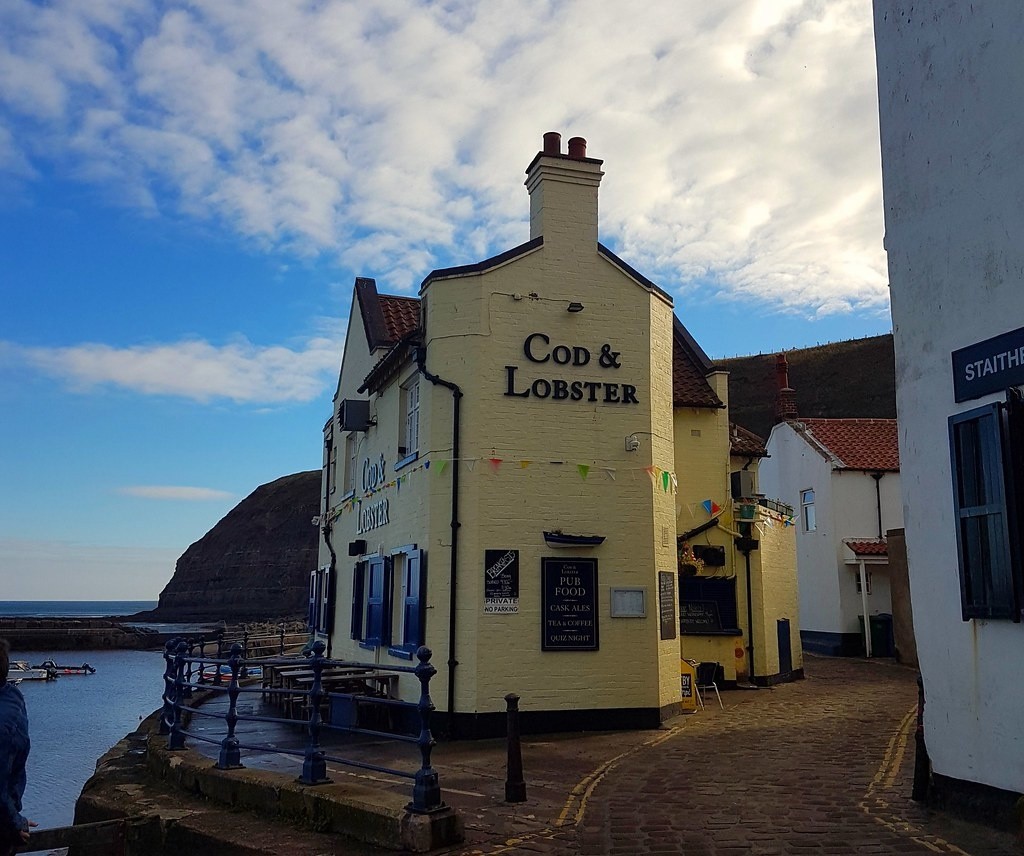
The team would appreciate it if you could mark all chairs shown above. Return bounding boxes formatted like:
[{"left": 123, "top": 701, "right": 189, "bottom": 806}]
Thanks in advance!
[{"left": 693, "top": 661, "right": 725, "bottom": 711}]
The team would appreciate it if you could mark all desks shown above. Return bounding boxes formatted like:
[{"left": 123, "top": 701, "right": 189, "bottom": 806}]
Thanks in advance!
[
  {"left": 295, "top": 670, "right": 400, "bottom": 737},
  {"left": 262, "top": 663, "right": 280, "bottom": 700},
  {"left": 273, "top": 664, "right": 311, "bottom": 705},
  {"left": 279, "top": 667, "right": 372, "bottom": 719}
]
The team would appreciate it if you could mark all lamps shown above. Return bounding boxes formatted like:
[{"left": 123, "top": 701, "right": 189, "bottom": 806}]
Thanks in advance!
[{"left": 567, "top": 301, "right": 584, "bottom": 313}]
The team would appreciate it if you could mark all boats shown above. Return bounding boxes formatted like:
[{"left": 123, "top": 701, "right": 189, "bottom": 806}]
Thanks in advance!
[
  {"left": 199, "top": 666, "right": 263, "bottom": 682},
  {"left": 7, "top": 663, "right": 59, "bottom": 685},
  {"left": 7, "top": 658, "right": 96, "bottom": 676}
]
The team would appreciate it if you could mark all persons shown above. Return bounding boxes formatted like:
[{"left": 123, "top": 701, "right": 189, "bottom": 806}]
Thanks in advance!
[{"left": 0, "top": 640, "right": 38, "bottom": 856}]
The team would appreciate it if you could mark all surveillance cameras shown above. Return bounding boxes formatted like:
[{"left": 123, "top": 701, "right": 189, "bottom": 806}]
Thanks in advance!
[{"left": 631, "top": 441, "right": 640, "bottom": 449}]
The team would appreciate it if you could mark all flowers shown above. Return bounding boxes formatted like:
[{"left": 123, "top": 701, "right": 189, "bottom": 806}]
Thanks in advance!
[{"left": 681, "top": 551, "right": 705, "bottom": 574}]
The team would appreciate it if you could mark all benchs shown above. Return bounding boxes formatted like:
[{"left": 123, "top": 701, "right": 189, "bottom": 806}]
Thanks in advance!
[{"left": 258, "top": 674, "right": 403, "bottom": 710}]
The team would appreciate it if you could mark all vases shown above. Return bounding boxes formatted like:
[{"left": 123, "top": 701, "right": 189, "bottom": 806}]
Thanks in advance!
[{"left": 684, "top": 564, "right": 698, "bottom": 576}]
